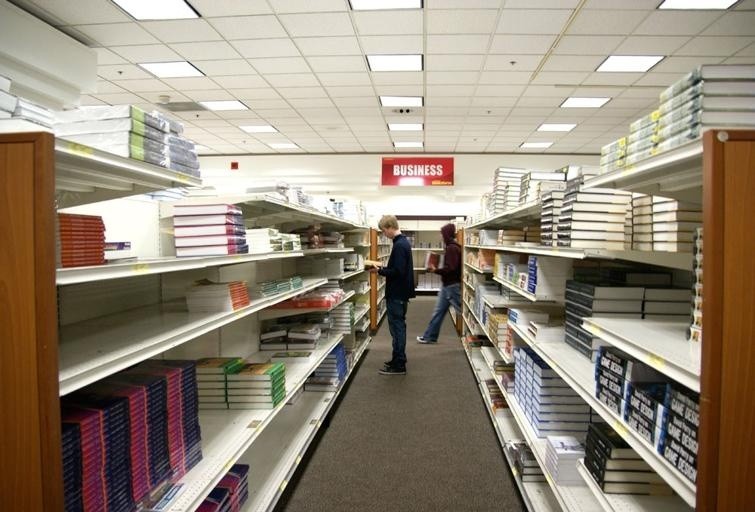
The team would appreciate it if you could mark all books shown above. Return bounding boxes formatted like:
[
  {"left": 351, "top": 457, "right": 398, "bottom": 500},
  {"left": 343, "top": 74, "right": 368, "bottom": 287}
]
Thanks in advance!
[
  {"left": 632, "top": 194, "right": 652, "bottom": 250},
  {"left": 564, "top": 260, "right": 690, "bottom": 364},
  {"left": 244, "top": 227, "right": 302, "bottom": 256},
  {"left": 557, "top": 188, "right": 634, "bottom": 250},
  {"left": 685, "top": 225, "right": 703, "bottom": 345},
  {"left": 546, "top": 435, "right": 585, "bottom": 488},
  {"left": 491, "top": 252, "right": 572, "bottom": 298},
  {"left": 59, "top": 358, "right": 204, "bottom": 511},
  {"left": 56, "top": 213, "right": 139, "bottom": 269},
  {"left": 581, "top": 424, "right": 673, "bottom": 495},
  {"left": 462, "top": 287, "right": 563, "bottom": 346},
  {"left": 488, "top": 166, "right": 565, "bottom": 249},
  {"left": 364, "top": 260, "right": 383, "bottom": 272},
  {"left": 173, "top": 204, "right": 249, "bottom": 259},
  {"left": 506, "top": 439, "right": 547, "bottom": 482},
  {"left": 554, "top": 167, "right": 598, "bottom": 189},
  {"left": 654, "top": 65, "right": 754, "bottom": 153},
  {"left": 295, "top": 218, "right": 367, "bottom": 300},
  {"left": 194, "top": 464, "right": 249, "bottom": 512},
  {"left": 425, "top": 250, "right": 444, "bottom": 273},
  {"left": 468, "top": 348, "right": 516, "bottom": 412},
  {"left": 465, "top": 214, "right": 492, "bottom": 287},
  {"left": 512, "top": 346, "right": 606, "bottom": 438},
  {"left": 594, "top": 345, "right": 632, "bottom": 420},
  {"left": 624, "top": 379, "right": 699, "bottom": 487},
  {"left": 596, "top": 137, "right": 623, "bottom": 174},
  {"left": 260, "top": 301, "right": 356, "bottom": 392},
  {"left": 197, "top": 355, "right": 286, "bottom": 410},
  {"left": 625, "top": 109, "right": 659, "bottom": 165},
  {"left": 650, "top": 193, "right": 702, "bottom": 251},
  {"left": 186, "top": 263, "right": 301, "bottom": 313},
  {"left": 1, "top": 73, "right": 200, "bottom": 182}
]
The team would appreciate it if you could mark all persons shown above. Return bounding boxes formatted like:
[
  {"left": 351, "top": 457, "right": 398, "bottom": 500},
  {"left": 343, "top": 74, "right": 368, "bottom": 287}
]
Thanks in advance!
[
  {"left": 368, "top": 214, "right": 418, "bottom": 374},
  {"left": 417, "top": 224, "right": 461, "bottom": 344}
]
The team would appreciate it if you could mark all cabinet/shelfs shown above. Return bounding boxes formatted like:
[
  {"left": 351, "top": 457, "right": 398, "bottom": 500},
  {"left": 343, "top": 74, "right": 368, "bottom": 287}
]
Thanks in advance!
[
  {"left": 0, "top": 132, "right": 373, "bottom": 512},
  {"left": 372, "top": 229, "right": 393, "bottom": 333},
  {"left": 448, "top": 227, "right": 461, "bottom": 336},
  {"left": 400, "top": 229, "right": 446, "bottom": 296},
  {"left": 462, "top": 129, "right": 755, "bottom": 512}
]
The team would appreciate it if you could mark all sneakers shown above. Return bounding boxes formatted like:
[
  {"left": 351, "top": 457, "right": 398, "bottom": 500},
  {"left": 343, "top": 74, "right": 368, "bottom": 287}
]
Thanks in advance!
[
  {"left": 417, "top": 335, "right": 438, "bottom": 344},
  {"left": 384, "top": 358, "right": 408, "bottom": 365},
  {"left": 378, "top": 367, "right": 407, "bottom": 374}
]
